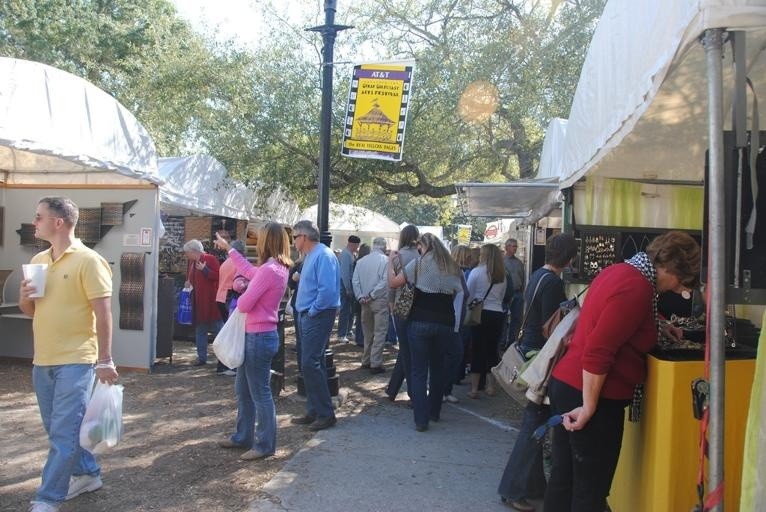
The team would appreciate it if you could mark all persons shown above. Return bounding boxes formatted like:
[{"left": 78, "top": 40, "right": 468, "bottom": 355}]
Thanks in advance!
[
  {"left": 544, "top": 230, "right": 701, "bottom": 512},
  {"left": 497, "top": 233, "right": 579, "bottom": 511},
  {"left": 213, "top": 221, "right": 294, "bottom": 461},
  {"left": 388, "top": 233, "right": 462, "bottom": 430},
  {"left": 657, "top": 269, "right": 707, "bottom": 328},
  {"left": 18, "top": 194, "right": 119, "bottom": 512},
  {"left": 183, "top": 238, "right": 225, "bottom": 367},
  {"left": 290, "top": 220, "right": 340, "bottom": 431},
  {"left": 288, "top": 219, "right": 525, "bottom": 410},
  {"left": 215, "top": 240, "right": 247, "bottom": 377}
]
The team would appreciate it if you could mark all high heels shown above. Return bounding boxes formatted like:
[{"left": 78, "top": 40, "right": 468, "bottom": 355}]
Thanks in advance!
[{"left": 501, "top": 496, "right": 536, "bottom": 511}]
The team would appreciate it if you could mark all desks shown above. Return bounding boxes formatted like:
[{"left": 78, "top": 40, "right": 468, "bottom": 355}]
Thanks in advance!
[{"left": 603, "top": 338, "right": 756, "bottom": 510}]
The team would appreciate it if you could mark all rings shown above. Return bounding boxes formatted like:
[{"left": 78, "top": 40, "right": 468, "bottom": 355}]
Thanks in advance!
[{"left": 571, "top": 428, "right": 573, "bottom": 433}]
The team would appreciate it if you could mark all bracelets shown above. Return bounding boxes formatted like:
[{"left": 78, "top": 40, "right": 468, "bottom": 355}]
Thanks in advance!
[{"left": 97, "top": 358, "right": 113, "bottom": 363}]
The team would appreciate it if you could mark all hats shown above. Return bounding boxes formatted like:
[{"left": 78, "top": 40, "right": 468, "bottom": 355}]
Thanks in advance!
[{"left": 348, "top": 236, "right": 361, "bottom": 244}]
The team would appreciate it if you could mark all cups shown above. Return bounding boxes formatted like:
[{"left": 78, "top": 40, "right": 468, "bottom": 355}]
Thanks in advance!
[{"left": 22, "top": 263, "right": 48, "bottom": 298}]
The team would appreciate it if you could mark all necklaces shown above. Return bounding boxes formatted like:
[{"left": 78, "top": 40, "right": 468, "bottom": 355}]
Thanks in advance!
[{"left": 678, "top": 279, "right": 695, "bottom": 301}]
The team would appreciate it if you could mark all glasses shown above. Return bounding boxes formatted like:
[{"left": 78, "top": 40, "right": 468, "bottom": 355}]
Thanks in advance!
[
  {"left": 37, "top": 214, "right": 59, "bottom": 221},
  {"left": 534, "top": 415, "right": 562, "bottom": 440},
  {"left": 292, "top": 234, "right": 302, "bottom": 240}
]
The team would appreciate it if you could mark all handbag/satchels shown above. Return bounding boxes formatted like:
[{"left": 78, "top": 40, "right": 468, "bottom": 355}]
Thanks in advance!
[
  {"left": 491, "top": 341, "right": 529, "bottom": 408},
  {"left": 393, "top": 282, "right": 415, "bottom": 320},
  {"left": 178, "top": 288, "right": 194, "bottom": 325},
  {"left": 462, "top": 298, "right": 483, "bottom": 326},
  {"left": 542, "top": 299, "right": 576, "bottom": 339},
  {"left": 224, "top": 289, "right": 239, "bottom": 318}
]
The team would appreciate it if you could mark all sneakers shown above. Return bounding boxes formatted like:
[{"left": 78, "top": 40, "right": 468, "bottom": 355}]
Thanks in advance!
[
  {"left": 217, "top": 440, "right": 261, "bottom": 460},
  {"left": 293, "top": 416, "right": 336, "bottom": 430},
  {"left": 217, "top": 370, "right": 236, "bottom": 376},
  {"left": 65, "top": 476, "right": 103, "bottom": 500},
  {"left": 30, "top": 501, "right": 62, "bottom": 512},
  {"left": 338, "top": 337, "right": 479, "bottom": 432}
]
